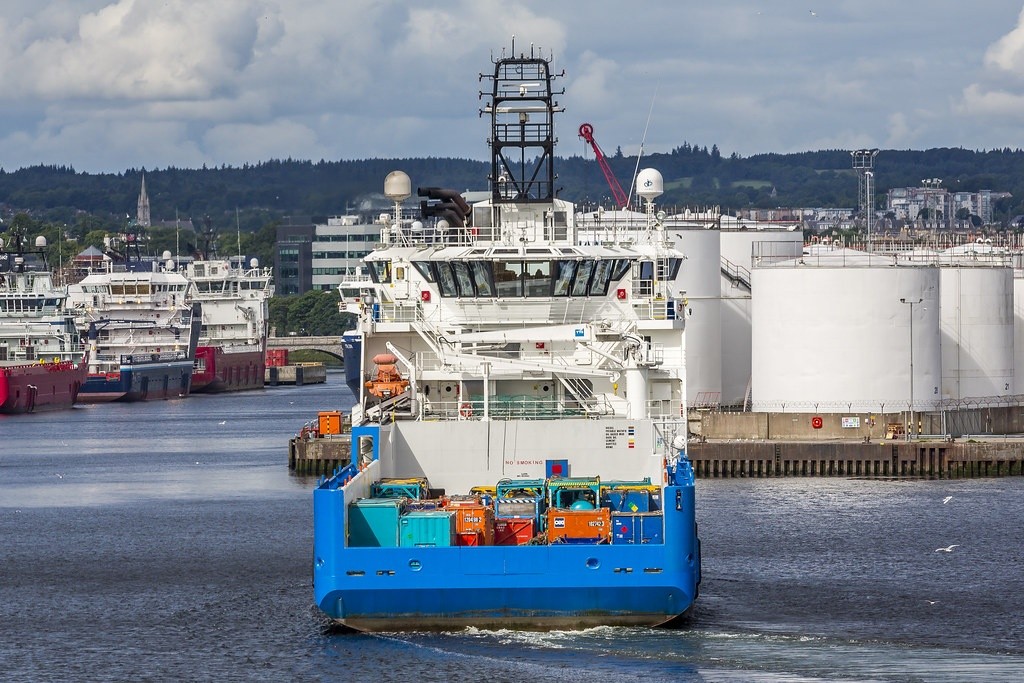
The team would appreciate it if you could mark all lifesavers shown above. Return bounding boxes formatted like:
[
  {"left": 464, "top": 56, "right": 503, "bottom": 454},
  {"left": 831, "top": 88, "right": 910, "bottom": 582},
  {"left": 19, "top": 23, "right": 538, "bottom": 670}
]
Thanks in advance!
[
  {"left": 459, "top": 403, "right": 474, "bottom": 418},
  {"left": 812, "top": 418, "right": 822, "bottom": 430}
]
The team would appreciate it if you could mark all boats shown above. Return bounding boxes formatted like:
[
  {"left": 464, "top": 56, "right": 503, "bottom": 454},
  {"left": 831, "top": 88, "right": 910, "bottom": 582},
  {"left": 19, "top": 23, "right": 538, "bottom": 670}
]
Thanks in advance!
[
  {"left": 312, "top": 169, "right": 700, "bottom": 630},
  {"left": 0, "top": 222, "right": 275, "bottom": 416}
]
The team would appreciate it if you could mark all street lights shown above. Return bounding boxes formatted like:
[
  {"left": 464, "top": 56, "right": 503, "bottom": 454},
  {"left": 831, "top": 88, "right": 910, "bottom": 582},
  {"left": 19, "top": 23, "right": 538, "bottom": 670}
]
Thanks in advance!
[
  {"left": 865, "top": 171, "right": 875, "bottom": 267},
  {"left": 899, "top": 298, "right": 924, "bottom": 437}
]
